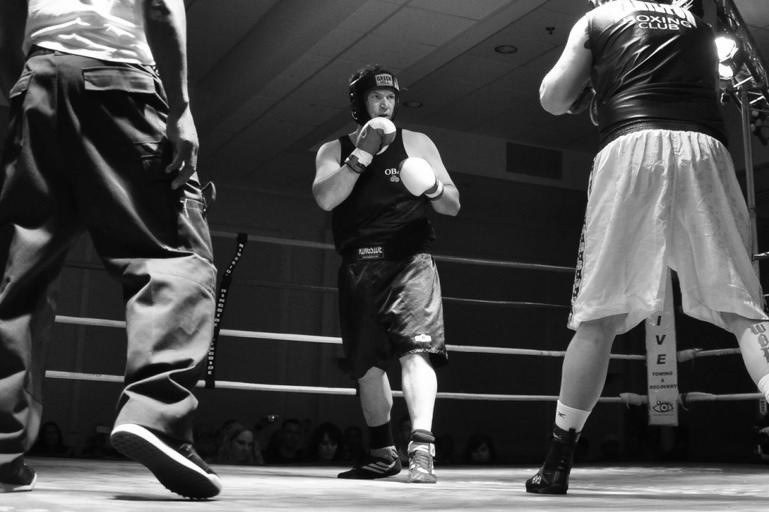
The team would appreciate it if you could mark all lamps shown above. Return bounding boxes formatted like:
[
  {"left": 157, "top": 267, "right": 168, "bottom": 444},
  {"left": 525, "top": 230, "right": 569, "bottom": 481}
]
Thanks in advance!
[{"left": 713, "top": 20, "right": 743, "bottom": 82}]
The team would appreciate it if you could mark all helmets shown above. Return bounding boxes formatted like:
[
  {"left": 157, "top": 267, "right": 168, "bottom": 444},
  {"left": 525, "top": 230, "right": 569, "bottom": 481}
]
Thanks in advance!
[{"left": 348, "top": 69, "right": 403, "bottom": 126}]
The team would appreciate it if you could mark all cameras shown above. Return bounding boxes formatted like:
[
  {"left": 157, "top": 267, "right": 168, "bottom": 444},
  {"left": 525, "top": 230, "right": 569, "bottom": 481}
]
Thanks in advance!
[{"left": 265, "top": 412, "right": 280, "bottom": 424}]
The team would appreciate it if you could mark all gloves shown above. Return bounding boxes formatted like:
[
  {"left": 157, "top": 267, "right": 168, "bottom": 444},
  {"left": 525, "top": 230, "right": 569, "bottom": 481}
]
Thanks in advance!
[
  {"left": 342, "top": 117, "right": 397, "bottom": 175},
  {"left": 397, "top": 157, "right": 444, "bottom": 202}
]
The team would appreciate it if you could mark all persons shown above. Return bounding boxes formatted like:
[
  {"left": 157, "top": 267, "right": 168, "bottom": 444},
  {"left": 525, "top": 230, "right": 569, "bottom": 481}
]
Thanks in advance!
[
  {"left": 524, "top": 2, "right": 769, "bottom": 498},
  {"left": 308, "top": 63, "right": 464, "bottom": 483},
  {"left": 32, "top": 410, "right": 514, "bottom": 466},
  {"left": 1, "top": 0, "right": 224, "bottom": 500}
]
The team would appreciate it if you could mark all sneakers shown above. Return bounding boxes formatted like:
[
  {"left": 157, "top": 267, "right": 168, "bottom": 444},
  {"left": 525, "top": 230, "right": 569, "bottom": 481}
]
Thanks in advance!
[
  {"left": 526, "top": 463, "right": 571, "bottom": 494},
  {"left": 338, "top": 443, "right": 401, "bottom": 479},
  {"left": 109, "top": 422, "right": 223, "bottom": 499},
  {"left": 407, "top": 440, "right": 438, "bottom": 484}
]
